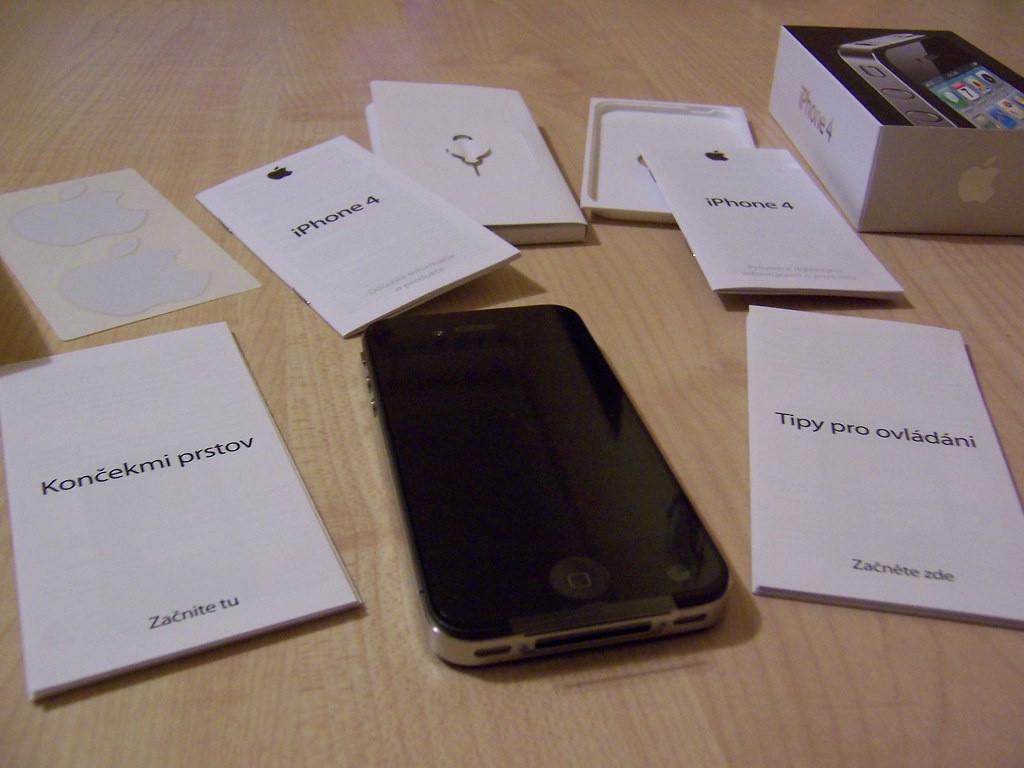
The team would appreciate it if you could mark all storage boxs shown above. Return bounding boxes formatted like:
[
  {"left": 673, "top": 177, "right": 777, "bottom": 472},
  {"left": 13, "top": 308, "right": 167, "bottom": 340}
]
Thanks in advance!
[{"left": 766, "top": 23, "right": 1024, "bottom": 237}]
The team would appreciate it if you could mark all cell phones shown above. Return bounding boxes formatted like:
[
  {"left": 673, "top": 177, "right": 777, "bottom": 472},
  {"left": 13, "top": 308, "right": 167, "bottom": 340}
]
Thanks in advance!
[
  {"left": 360, "top": 306, "right": 729, "bottom": 666},
  {"left": 839, "top": 32, "right": 1024, "bottom": 130}
]
